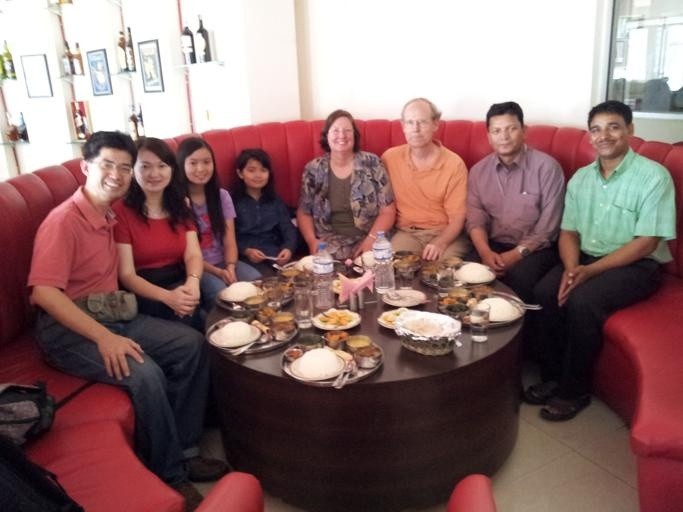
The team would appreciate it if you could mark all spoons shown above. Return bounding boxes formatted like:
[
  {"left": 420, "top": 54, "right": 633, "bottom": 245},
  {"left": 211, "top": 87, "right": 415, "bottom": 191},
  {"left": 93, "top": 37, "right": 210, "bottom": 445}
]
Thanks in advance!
[
  {"left": 230, "top": 333, "right": 272, "bottom": 358},
  {"left": 333, "top": 357, "right": 355, "bottom": 390}
]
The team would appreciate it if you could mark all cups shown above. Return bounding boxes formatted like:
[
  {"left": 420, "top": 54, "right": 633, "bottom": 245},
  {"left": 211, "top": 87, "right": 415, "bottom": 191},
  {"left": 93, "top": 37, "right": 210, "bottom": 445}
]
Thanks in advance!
[
  {"left": 361, "top": 250, "right": 372, "bottom": 273},
  {"left": 290, "top": 280, "right": 315, "bottom": 328},
  {"left": 467, "top": 300, "right": 489, "bottom": 343},
  {"left": 396, "top": 260, "right": 415, "bottom": 290}
]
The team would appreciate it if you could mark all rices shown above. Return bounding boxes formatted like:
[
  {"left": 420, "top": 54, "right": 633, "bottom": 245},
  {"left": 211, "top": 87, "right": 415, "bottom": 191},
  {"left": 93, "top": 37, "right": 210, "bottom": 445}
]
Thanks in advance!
[
  {"left": 216, "top": 322, "right": 252, "bottom": 344},
  {"left": 480, "top": 297, "right": 518, "bottom": 322},
  {"left": 455, "top": 263, "right": 491, "bottom": 283},
  {"left": 297, "top": 255, "right": 315, "bottom": 270},
  {"left": 223, "top": 282, "right": 257, "bottom": 301},
  {"left": 294, "top": 348, "right": 337, "bottom": 379}
]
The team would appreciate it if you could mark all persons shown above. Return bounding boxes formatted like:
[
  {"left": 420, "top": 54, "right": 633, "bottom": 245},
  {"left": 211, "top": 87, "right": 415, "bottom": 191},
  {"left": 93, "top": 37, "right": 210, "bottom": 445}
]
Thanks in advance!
[
  {"left": 108, "top": 136, "right": 205, "bottom": 339},
  {"left": 380, "top": 98, "right": 474, "bottom": 260},
  {"left": 174, "top": 138, "right": 263, "bottom": 311},
  {"left": 26, "top": 131, "right": 229, "bottom": 511},
  {"left": 464, "top": 102, "right": 564, "bottom": 299},
  {"left": 295, "top": 108, "right": 397, "bottom": 261},
  {"left": 522, "top": 100, "right": 680, "bottom": 423},
  {"left": 228, "top": 148, "right": 299, "bottom": 277}
]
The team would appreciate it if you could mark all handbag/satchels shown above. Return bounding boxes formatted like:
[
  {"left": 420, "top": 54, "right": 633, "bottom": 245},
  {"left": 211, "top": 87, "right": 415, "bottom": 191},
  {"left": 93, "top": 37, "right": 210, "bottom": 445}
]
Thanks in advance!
[{"left": 73, "top": 291, "right": 140, "bottom": 322}]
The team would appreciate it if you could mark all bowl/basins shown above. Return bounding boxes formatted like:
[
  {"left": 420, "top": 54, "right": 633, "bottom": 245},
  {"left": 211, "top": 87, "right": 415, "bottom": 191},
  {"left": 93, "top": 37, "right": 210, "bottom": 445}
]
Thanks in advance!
[
  {"left": 422, "top": 255, "right": 458, "bottom": 287},
  {"left": 344, "top": 334, "right": 371, "bottom": 353},
  {"left": 285, "top": 346, "right": 301, "bottom": 363},
  {"left": 390, "top": 249, "right": 419, "bottom": 271},
  {"left": 247, "top": 274, "right": 289, "bottom": 310},
  {"left": 320, "top": 330, "right": 346, "bottom": 352},
  {"left": 354, "top": 349, "right": 383, "bottom": 370},
  {"left": 299, "top": 335, "right": 324, "bottom": 349},
  {"left": 443, "top": 283, "right": 494, "bottom": 317},
  {"left": 227, "top": 310, "right": 299, "bottom": 335}
]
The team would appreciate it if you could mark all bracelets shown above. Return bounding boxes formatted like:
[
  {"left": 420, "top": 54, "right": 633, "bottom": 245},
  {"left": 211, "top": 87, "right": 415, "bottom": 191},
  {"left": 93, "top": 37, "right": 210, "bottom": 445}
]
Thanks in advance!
[
  {"left": 516, "top": 244, "right": 529, "bottom": 257},
  {"left": 186, "top": 272, "right": 202, "bottom": 279},
  {"left": 367, "top": 232, "right": 378, "bottom": 240},
  {"left": 223, "top": 261, "right": 236, "bottom": 266}
]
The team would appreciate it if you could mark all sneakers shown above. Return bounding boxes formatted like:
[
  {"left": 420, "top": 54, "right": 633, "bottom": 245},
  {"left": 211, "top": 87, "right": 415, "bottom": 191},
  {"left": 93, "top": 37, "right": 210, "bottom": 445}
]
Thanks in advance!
[
  {"left": 173, "top": 481, "right": 203, "bottom": 512},
  {"left": 198, "top": 459, "right": 229, "bottom": 482}
]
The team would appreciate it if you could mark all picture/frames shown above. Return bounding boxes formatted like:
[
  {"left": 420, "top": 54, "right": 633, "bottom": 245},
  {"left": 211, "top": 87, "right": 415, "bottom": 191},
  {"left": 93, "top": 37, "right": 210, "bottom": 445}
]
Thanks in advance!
[
  {"left": 16, "top": 51, "right": 53, "bottom": 101},
  {"left": 69, "top": 101, "right": 94, "bottom": 141},
  {"left": 133, "top": 37, "right": 166, "bottom": 95},
  {"left": 85, "top": 46, "right": 115, "bottom": 101}
]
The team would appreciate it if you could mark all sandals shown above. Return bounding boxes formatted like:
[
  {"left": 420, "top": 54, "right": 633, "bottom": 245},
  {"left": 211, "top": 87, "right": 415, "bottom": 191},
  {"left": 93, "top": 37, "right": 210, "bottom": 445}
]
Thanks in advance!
[{"left": 526, "top": 380, "right": 591, "bottom": 421}]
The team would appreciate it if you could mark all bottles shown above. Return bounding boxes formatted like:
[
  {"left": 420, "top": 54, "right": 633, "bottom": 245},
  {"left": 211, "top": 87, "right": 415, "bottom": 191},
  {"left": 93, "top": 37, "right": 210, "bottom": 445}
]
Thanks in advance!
[
  {"left": 0, "top": 108, "right": 28, "bottom": 143},
  {"left": 61, "top": 39, "right": 82, "bottom": 76},
  {"left": 178, "top": 9, "right": 216, "bottom": 64},
  {"left": 347, "top": 288, "right": 366, "bottom": 312},
  {"left": 115, "top": 26, "right": 138, "bottom": 71},
  {"left": 127, "top": 104, "right": 145, "bottom": 140},
  {"left": 0, "top": 39, "right": 16, "bottom": 82},
  {"left": 71, "top": 98, "right": 87, "bottom": 145},
  {"left": 372, "top": 231, "right": 393, "bottom": 295},
  {"left": 312, "top": 244, "right": 337, "bottom": 310}
]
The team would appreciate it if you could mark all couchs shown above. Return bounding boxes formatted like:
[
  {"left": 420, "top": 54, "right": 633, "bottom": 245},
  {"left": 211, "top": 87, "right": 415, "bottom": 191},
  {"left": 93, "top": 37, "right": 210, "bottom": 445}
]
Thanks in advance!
[{"left": 1, "top": 113, "right": 682, "bottom": 512}]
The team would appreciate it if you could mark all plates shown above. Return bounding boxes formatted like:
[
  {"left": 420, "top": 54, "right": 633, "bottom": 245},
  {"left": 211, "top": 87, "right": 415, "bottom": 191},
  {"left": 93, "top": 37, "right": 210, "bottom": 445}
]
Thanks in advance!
[
  {"left": 209, "top": 324, "right": 257, "bottom": 350},
  {"left": 289, "top": 352, "right": 346, "bottom": 382},
  {"left": 477, "top": 300, "right": 524, "bottom": 323},
  {"left": 294, "top": 255, "right": 316, "bottom": 271},
  {"left": 355, "top": 255, "right": 365, "bottom": 265},
  {"left": 218, "top": 289, "right": 264, "bottom": 303},
  {"left": 455, "top": 265, "right": 497, "bottom": 282},
  {"left": 310, "top": 312, "right": 361, "bottom": 332},
  {"left": 375, "top": 309, "right": 408, "bottom": 330},
  {"left": 379, "top": 288, "right": 428, "bottom": 306}
]
